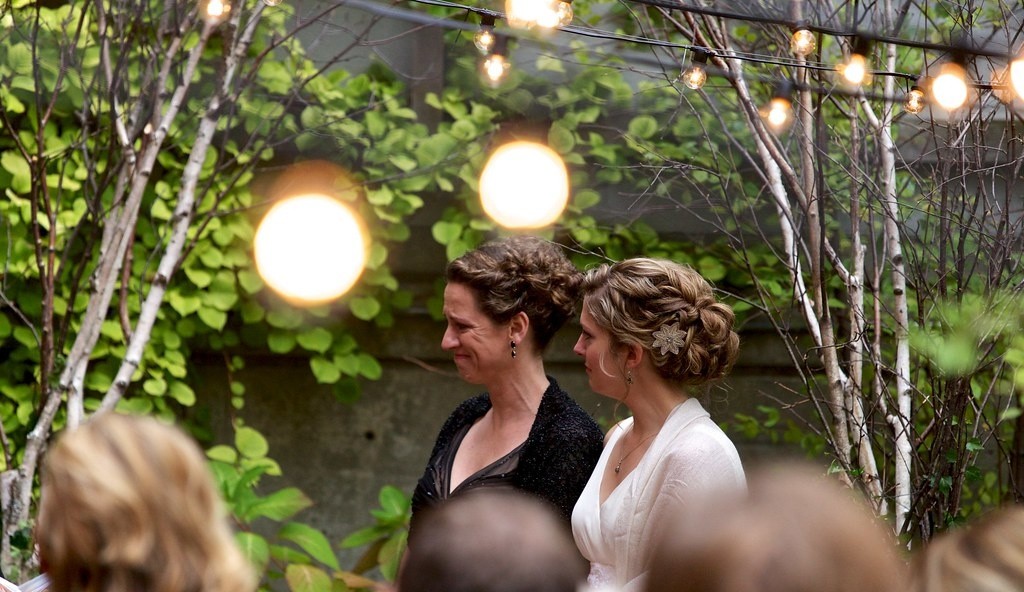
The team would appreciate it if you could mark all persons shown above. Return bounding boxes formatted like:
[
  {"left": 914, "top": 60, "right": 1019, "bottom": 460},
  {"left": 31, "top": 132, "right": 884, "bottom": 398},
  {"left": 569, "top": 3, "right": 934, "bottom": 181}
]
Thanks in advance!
[
  {"left": 642, "top": 462, "right": 909, "bottom": 592},
  {"left": 19, "top": 413, "right": 260, "bottom": 592},
  {"left": 393, "top": 487, "right": 588, "bottom": 592},
  {"left": 570, "top": 257, "right": 752, "bottom": 592},
  {"left": 407, "top": 234, "right": 609, "bottom": 552},
  {"left": 910, "top": 504, "right": 1024, "bottom": 592}
]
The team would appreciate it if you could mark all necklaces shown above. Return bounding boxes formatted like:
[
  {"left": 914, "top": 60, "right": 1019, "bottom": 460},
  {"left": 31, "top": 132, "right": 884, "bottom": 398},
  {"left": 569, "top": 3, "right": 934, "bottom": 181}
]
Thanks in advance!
[{"left": 614, "top": 422, "right": 659, "bottom": 473}]
